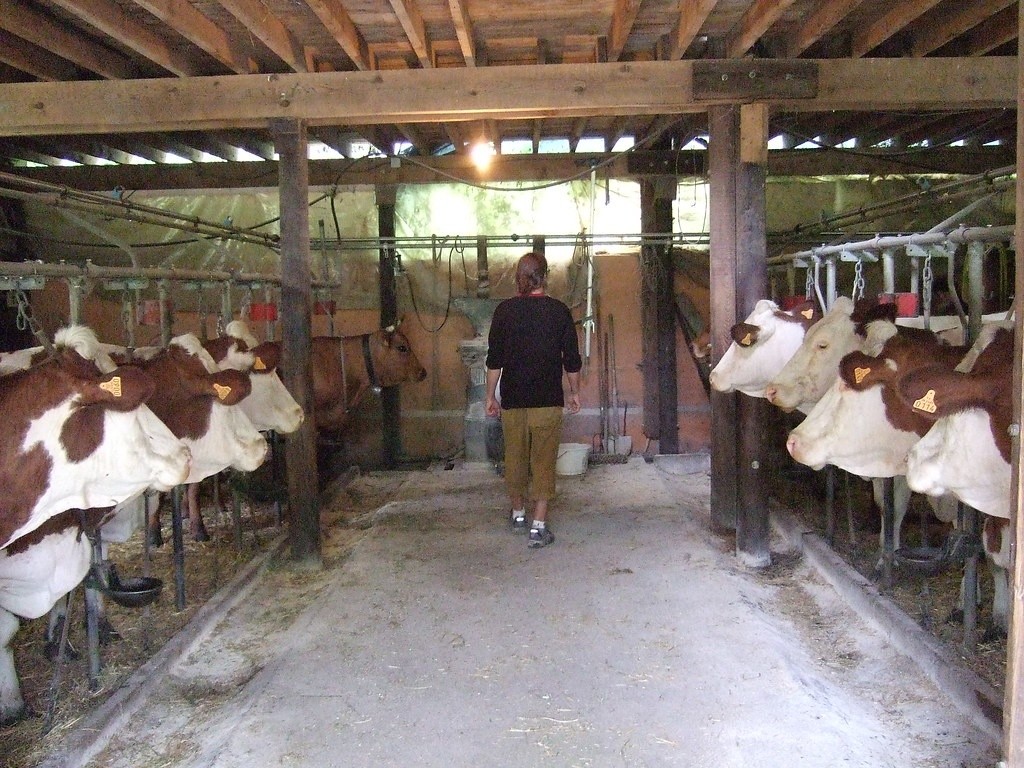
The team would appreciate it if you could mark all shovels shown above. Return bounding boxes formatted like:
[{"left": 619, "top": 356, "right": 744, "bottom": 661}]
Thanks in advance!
[{"left": 602, "top": 313, "right": 632, "bottom": 456}]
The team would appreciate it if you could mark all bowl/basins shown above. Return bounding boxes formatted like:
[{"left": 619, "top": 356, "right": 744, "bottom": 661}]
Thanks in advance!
[
  {"left": 893, "top": 546, "right": 944, "bottom": 575},
  {"left": 112, "top": 576, "right": 163, "bottom": 607}
]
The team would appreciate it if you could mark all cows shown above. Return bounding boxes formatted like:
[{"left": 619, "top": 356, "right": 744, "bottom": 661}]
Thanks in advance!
[
  {"left": 0, "top": 315, "right": 429, "bottom": 723},
  {"left": 707, "top": 294, "right": 1016, "bottom": 647}
]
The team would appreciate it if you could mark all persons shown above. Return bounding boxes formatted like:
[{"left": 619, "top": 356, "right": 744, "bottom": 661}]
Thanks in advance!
[{"left": 483, "top": 253, "right": 582, "bottom": 549}]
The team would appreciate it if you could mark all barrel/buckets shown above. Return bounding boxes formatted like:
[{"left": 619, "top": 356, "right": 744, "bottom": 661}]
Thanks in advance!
[{"left": 555, "top": 442, "right": 591, "bottom": 476}]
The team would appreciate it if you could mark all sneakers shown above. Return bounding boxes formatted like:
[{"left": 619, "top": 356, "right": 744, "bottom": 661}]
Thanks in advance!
[
  {"left": 509, "top": 507, "right": 527, "bottom": 535},
  {"left": 528, "top": 528, "right": 554, "bottom": 548}
]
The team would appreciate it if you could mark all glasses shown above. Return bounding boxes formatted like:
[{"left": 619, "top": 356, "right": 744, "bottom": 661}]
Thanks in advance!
[{"left": 544, "top": 268, "right": 551, "bottom": 276}]
